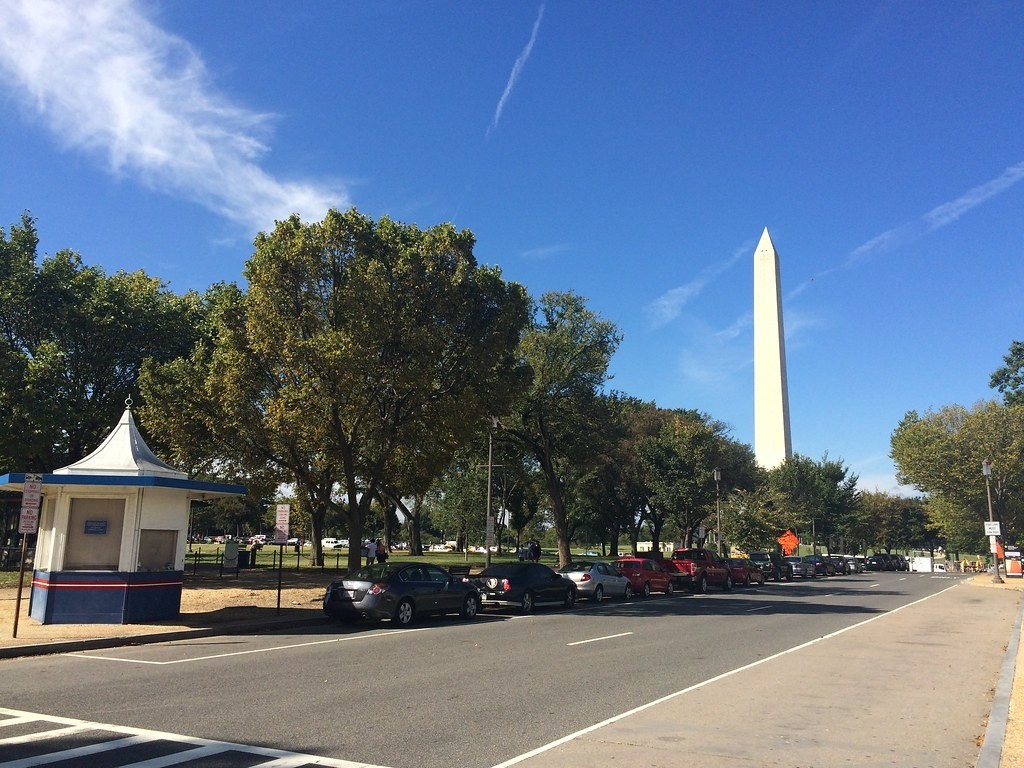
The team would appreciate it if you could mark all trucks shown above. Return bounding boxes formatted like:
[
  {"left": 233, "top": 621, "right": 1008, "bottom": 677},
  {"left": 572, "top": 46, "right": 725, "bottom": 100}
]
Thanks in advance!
[{"left": 905, "top": 556, "right": 946, "bottom": 573}]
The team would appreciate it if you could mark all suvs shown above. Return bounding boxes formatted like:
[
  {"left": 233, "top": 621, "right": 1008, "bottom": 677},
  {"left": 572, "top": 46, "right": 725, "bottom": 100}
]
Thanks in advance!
[
  {"left": 873, "top": 554, "right": 909, "bottom": 572},
  {"left": 579, "top": 551, "right": 601, "bottom": 556},
  {"left": 750, "top": 551, "right": 793, "bottom": 582},
  {"left": 668, "top": 547, "right": 733, "bottom": 594}
]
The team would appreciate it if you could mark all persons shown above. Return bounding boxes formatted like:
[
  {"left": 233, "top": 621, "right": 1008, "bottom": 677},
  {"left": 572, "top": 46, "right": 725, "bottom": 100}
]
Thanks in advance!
[
  {"left": 518, "top": 542, "right": 541, "bottom": 563},
  {"left": 366, "top": 539, "right": 377, "bottom": 566},
  {"left": 376, "top": 539, "right": 385, "bottom": 564}
]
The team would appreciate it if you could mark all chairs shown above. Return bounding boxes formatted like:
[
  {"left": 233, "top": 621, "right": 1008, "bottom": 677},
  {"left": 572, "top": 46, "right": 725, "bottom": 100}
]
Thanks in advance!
[{"left": 410, "top": 570, "right": 421, "bottom": 581}]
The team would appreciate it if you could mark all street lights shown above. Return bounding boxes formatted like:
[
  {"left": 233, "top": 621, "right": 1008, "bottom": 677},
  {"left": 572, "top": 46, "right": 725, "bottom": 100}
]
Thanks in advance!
[{"left": 713, "top": 466, "right": 722, "bottom": 556}]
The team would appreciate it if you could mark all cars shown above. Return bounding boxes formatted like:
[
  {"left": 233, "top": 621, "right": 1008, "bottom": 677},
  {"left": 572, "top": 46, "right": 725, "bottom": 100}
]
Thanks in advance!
[
  {"left": 847, "top": 558, "right": 863, "bottom": 572},
  {"left": 725, "top": 558, "right": 765, "bottom": 587},
  {"left": 866, "top": 556, "right": 885, "bottom": 571},
  {"left": 554, "top": 560, "right": 633, "bottom": 604},
  {"left": 784, "top": 556, "right": 815, "bottom": 578},
  {"left": 471, "top": 546, "right": 558, "bottom": 557},
  {"left": 463, "top": 561, "right": 577, "bottom": 613},
  {"left": 826, "top": 555, "right": 851, "bottom": 575},
  {"left": 613, "top": 559, "right": 674, "bottom": 599},
  {"left": 288, "top": 537, "right": 383, "bottom": 549},
  {"left": 188, "top": 534, "right": 274, "bottom": 544},
  {"left": 323, "top": 561, "right": 482, "bottom": 628},
  {"left": 805, "top": 555, "right": 836, "bottom": 576}
]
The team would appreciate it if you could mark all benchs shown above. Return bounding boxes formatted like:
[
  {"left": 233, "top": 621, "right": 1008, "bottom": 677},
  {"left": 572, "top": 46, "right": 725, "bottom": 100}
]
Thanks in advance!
[{"left": 449, "top": 565, "right": 471, "bottom": 576}]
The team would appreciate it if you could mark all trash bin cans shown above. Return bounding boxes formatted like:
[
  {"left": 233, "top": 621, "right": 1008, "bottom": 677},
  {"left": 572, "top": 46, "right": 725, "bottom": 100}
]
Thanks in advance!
[
  {"left": 295, "top": 544, "right": 300, "bottom": 552},
  {"left": 238, "top": 550, "right": 251, "bottom": 569}
]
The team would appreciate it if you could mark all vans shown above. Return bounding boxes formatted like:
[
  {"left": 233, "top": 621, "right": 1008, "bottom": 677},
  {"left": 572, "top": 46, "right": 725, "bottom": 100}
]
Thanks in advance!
[{"left": 429, "top": 544, "right": 452, "bottom": 553}]
